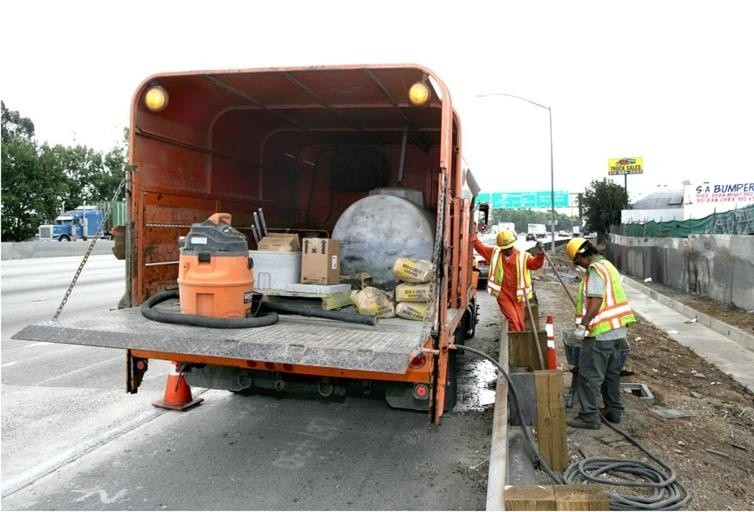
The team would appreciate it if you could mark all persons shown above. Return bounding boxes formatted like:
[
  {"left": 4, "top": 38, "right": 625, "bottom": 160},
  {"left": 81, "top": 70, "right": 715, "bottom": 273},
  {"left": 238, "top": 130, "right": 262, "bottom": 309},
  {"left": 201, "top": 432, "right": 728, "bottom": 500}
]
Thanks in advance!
[
  {"left": 471, "top": 222, "right": 545, "bottom": 332},
  {"left": 567, "top": 237, "right": 637, "bottom": 429}
]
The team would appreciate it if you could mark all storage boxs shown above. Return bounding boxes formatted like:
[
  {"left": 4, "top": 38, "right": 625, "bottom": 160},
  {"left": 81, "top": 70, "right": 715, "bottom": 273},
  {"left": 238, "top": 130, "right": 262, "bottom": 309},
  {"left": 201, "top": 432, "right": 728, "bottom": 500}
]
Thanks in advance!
[{"left": 256, "top": 232, "right": 341, "bottom": 286}]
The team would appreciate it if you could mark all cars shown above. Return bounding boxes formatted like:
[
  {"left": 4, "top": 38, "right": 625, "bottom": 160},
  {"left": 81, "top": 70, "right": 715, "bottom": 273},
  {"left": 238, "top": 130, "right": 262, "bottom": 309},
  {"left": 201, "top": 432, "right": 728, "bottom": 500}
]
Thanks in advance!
[{"left": 472, "top": 221, "right": 597, "bottom": 286}]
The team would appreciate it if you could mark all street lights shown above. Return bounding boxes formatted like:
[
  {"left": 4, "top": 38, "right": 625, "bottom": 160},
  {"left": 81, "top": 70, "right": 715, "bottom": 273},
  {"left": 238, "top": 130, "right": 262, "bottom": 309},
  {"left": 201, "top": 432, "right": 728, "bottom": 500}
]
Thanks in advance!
[{"left": 477, "top": 92, "right": 555, "bottom": 259}]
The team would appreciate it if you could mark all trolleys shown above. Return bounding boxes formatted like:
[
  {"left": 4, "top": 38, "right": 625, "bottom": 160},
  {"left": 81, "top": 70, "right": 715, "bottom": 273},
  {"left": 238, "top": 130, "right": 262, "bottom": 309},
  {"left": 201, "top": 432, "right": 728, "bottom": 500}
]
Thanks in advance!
[{"left": 561, "top": 327, "right": 630, "bottom": 411}]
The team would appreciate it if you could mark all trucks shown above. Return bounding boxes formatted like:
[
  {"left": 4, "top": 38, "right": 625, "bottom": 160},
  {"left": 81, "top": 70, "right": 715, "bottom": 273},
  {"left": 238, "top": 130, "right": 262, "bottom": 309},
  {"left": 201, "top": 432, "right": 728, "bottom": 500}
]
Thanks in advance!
[
  {"left": 35, "top": 199, "right": 125, "bottom": 243},
  {"left": 10, "top": 65, "right": 485, "bottom": 428}
]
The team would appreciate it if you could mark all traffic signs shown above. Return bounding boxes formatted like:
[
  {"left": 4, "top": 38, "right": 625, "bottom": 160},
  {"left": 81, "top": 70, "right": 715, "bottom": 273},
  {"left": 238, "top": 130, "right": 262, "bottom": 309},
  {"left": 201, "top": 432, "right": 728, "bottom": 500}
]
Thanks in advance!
[
  {"left": 473, "top": 192, "right": 490, "bottom": 209},
  {"left": 534, "top": 191, "right": 570, "bottom": 209},
  {"left": 491, "top": 191, "right": 536, "bottom": 208}
]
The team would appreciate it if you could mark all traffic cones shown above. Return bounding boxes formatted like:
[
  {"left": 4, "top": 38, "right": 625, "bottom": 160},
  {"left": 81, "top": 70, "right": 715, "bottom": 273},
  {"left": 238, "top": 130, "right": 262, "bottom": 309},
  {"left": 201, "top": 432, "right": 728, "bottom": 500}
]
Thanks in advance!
[
  {"left": 544, "top": 313, "right": 560, "bottom": 370},
  {"left": 148, "top": 358, "right": 206, "bottom": 411}
]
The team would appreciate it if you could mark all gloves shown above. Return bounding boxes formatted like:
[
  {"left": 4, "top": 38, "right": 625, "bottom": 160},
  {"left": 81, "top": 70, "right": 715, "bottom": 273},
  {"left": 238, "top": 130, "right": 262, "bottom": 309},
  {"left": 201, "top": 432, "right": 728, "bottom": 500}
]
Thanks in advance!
[
  {"left": 572, "top": 324, "right": 587, "bottom": 341},
  {"left": 534, "top": 241, "right": 545, "bottom": 255}
]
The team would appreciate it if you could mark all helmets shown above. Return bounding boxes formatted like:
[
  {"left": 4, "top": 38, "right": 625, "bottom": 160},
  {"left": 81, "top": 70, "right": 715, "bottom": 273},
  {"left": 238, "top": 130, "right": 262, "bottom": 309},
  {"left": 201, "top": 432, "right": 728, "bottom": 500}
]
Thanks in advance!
[
  {"left": 495, "top": 229, "right": 516, "bottom": 250},
  {"left": 564, "top": 236, "right": 589, "bottom": 265}
]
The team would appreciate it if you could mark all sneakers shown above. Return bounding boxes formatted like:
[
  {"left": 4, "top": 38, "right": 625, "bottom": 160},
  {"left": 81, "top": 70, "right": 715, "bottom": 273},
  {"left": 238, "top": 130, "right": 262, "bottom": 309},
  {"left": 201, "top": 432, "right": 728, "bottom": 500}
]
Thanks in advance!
[
  {"left": 598, "top": 406, "right": 622, "bottom": 425},
  {"left": 565, "top": 414, "right": 600, "bottom": 429}
]
